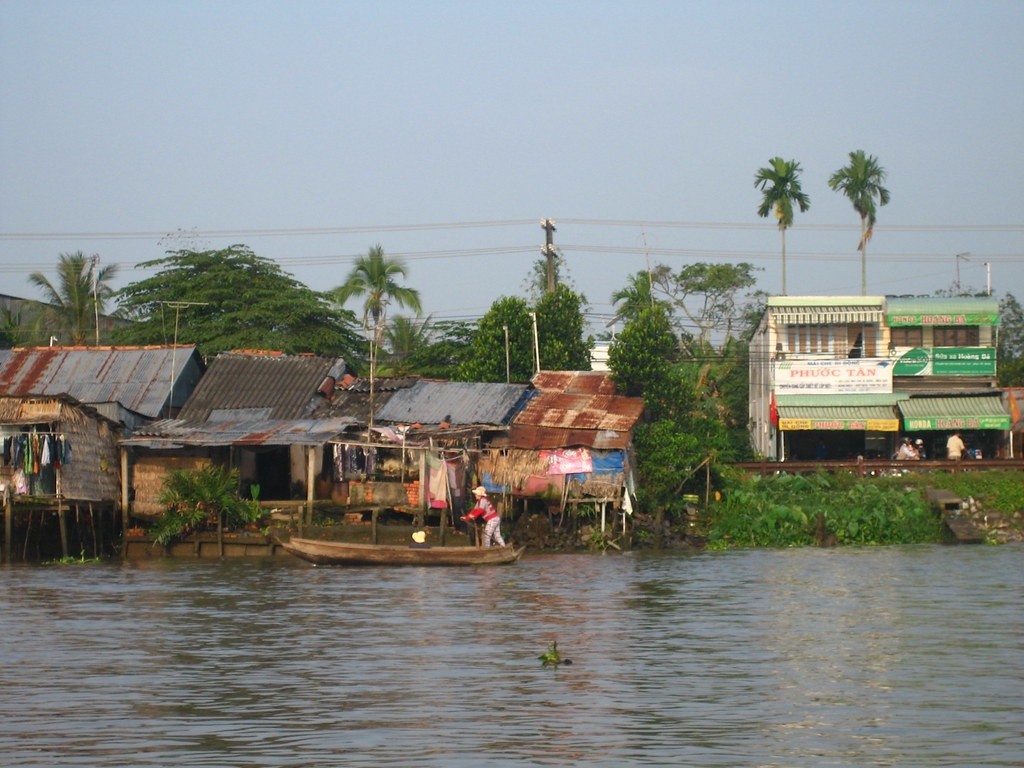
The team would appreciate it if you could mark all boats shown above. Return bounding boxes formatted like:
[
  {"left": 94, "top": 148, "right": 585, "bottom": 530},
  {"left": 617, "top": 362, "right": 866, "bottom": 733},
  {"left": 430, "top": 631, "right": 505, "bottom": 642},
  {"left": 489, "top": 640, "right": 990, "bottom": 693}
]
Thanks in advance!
[{"left": 270, "top": 531, "right": 530, "bottom": 566}]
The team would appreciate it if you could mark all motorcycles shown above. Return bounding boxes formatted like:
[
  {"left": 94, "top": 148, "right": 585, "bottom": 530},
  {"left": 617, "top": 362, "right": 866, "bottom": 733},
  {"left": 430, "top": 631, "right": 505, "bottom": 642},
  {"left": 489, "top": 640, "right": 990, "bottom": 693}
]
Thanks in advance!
[
  {"left": 890, "top": 436, "right": 927, "bottom": 460},
  {"left": 936, "top": 436, "right": 983, "bottom": 461}
]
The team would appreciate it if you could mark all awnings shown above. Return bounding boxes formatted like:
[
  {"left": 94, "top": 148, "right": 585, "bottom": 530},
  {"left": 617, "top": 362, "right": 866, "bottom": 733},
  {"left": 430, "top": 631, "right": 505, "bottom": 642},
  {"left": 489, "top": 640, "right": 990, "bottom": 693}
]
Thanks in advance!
[
  {"left": 776, "top": 406, "right": 899, "bottom": 432},
  {"left": 898, "top": 396, "right": 1011, "bottom": 431}
]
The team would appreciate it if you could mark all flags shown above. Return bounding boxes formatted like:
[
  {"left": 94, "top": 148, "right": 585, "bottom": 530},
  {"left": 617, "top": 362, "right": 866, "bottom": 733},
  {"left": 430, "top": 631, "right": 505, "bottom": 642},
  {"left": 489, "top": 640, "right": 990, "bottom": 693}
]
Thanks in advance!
[
  {"left": 770, "top": 393, "right": 777, "bottom": 426},
  {"left": 1008, "top": 390, "right": 1020, "bottom": 423}
]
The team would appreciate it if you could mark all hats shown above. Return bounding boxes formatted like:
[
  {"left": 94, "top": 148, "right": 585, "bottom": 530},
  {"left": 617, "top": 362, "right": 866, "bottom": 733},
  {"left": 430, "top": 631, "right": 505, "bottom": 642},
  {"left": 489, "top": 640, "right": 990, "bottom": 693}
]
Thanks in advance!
[
  {"left": 471, "top": 486, "right": 487, "bottom": 497},
  {"left": 412, "top": 530, "right": 426, "bottom": 543}
]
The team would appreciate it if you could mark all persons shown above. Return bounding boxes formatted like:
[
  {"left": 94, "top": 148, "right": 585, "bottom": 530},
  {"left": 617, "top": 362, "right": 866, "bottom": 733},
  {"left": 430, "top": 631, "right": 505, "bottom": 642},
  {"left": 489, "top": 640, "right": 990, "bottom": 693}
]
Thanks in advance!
[
  {"left": 460, "top": 486, "right": 505, "bottom": 548},
  {"left": 946, "top": 432, "right": 966, "bottom": 461},
  {"left": 815, "top": 437, "right": 827, "bottom": 459},
  {"left": 897, "top": 437, "right": 926, "bottom": 460}
]
